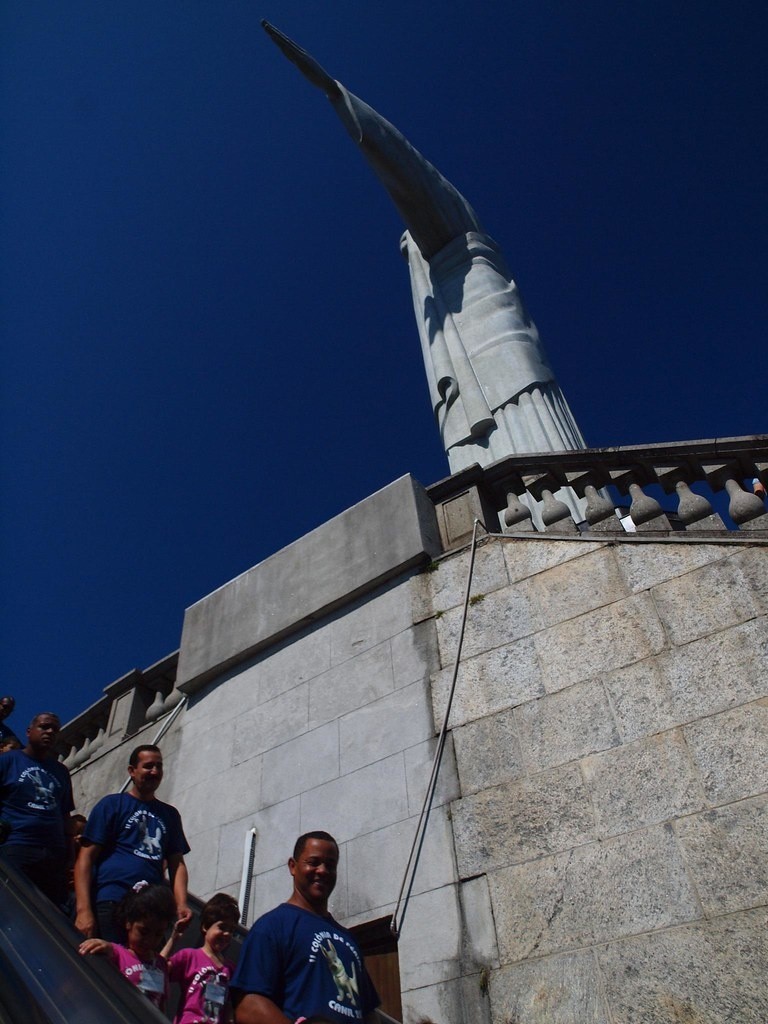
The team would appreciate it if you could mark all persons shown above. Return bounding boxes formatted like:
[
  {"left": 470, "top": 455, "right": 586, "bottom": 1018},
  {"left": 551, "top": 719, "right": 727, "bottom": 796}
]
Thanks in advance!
[
  {"left": 73, "top": 744, "right": 194, "bottom": 946},
  {"left": 0, "top": 735, "right": 22, "bottom": 755},
  {"left": 225, "top": 829, "right": 382, "bottom": 1024},
  {"left": 0, "top": 712, "right": 78, "bottom": 912},
  {"left": 156, "top": 894, "right": 242, "bottom": 1024},
  {"left": 76, "top": 882, "right": 183, "bottom": 1024},
  {"left": 257, "top": 21, "right": 605, "bottom": 525},
  {"left": 0, "top": 694, "right": 27, "bottom": 751},
  {"left": 71, "top": 814, "right": 86, "bottom": 849}
]
